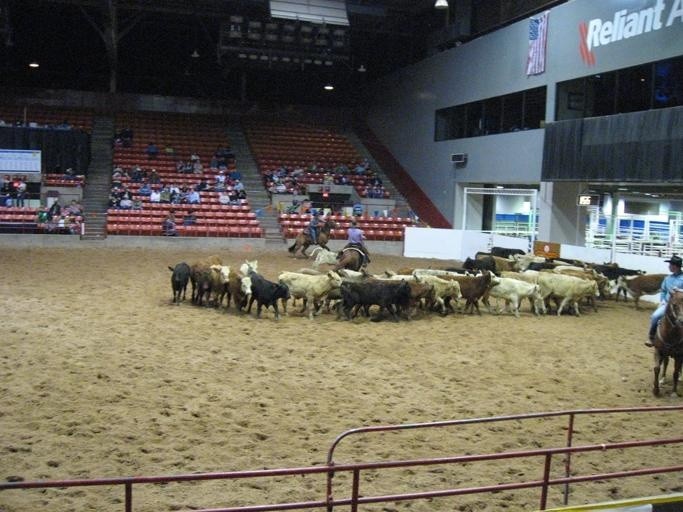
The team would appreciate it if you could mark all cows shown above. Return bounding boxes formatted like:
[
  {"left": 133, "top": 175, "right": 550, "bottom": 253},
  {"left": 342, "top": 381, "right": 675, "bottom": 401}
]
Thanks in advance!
[
  {"left": 166, "top": 255, "right": 292, "bottom": 321},
  {"left": 278, "top": 248, "right": 668, "bottom": 325}
]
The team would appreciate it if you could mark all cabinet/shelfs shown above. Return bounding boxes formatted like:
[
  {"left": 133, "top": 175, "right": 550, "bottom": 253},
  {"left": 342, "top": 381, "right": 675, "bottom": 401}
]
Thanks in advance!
[{"left": 494, "top": 220, "right": 683, "bottom": 262}]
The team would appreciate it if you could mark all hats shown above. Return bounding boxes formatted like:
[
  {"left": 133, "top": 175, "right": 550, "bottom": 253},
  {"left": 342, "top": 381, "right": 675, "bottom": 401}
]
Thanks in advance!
[{"left": 664, "top": 256, "right": 683, "bottom": 267}]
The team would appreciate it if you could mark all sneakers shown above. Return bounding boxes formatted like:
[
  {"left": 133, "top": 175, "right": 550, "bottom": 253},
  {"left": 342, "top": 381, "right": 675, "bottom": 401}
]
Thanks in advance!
[{"left": 645, "top": 336, "right": 657, "bottom": 346}]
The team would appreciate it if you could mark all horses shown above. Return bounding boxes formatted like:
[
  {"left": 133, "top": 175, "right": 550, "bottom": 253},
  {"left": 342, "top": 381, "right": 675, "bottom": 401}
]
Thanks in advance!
[
  {"left": 653, "top": 290, "right": 683, "bottom": 399},
  {"left": 287, "top": 218, "right": 341, "bottom": 259},
  {"left": 335, "top": 248, "right": 362, "bottom": 271}
]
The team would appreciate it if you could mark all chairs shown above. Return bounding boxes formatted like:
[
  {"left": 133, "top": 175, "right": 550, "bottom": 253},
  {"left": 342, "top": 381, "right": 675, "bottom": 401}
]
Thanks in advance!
[
  {"left": 103, "top": 110, "right": 262, "bottom": 241},
  {"left": 236, "top": 114, "right": 419, "bottom": 244},
  {"left": 0, "top": 171, "right": 85, "bottom": 234},
  {"left": 0, "top": 107, "right": 96, "bottom": 137}
]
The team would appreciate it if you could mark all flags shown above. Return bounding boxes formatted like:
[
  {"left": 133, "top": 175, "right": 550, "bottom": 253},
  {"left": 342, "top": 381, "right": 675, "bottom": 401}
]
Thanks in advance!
[{"left": 525, "top": 13, "right": 548, "bottom": 76}]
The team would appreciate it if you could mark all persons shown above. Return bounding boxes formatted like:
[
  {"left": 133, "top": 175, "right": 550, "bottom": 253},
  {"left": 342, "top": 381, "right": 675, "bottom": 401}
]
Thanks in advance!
[
  {"left": 1, "top": 168, "right": 85, "bottom": 234},
  {"left": 336, "top": 222, "right": 371, "bottom": 262},
  {"left": 309, "top": 212, "right": 327, "bottom": 244},
  {"left": 267, "top": 159, "right": 416, "bottom": 219},
  {"left": 644, "top": 254, "right": 683, "bottom": 346},
  {"left": 108, "top": 125, "right": 248, "bottom": 236}
]
